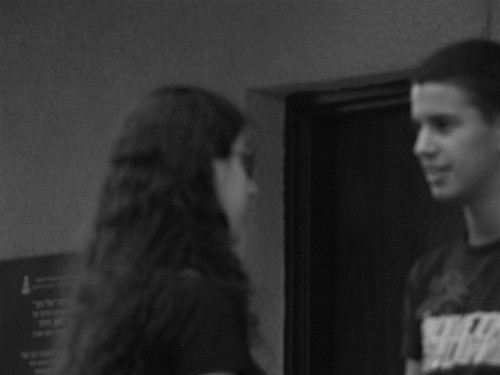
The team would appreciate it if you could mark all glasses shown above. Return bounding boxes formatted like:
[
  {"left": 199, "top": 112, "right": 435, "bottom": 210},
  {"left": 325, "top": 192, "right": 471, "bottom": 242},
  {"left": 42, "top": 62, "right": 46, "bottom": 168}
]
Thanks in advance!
[{"left": 231, "top": 145, "right": 254, "bottom": 176}]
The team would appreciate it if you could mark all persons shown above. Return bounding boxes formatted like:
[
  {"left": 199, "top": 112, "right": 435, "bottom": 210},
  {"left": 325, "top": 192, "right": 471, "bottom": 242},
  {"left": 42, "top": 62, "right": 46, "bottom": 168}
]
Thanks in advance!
[
  {"left": 40, "top": 84, "right": 271, "bottom": 375},
  {"left": 397, "top": 39, "right": 500, "bottom": 375}
]
